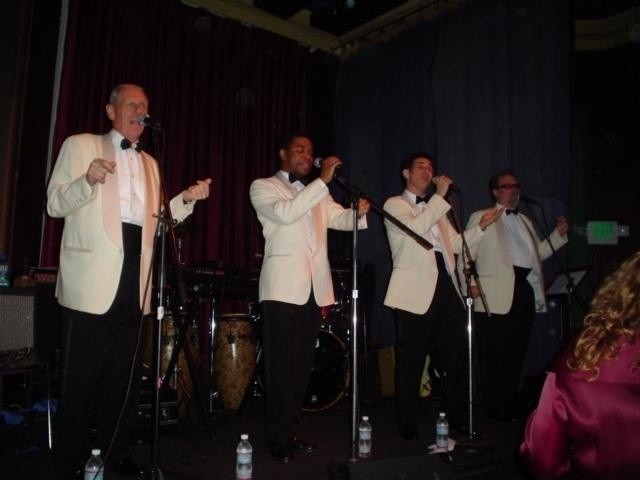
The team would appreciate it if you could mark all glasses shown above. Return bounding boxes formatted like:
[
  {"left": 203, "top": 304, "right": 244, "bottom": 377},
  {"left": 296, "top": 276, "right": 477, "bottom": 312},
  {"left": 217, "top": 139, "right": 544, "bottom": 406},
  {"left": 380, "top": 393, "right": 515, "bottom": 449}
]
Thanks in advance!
[{"left": 498, "top": 183, "right": 521, "bottom": 189}]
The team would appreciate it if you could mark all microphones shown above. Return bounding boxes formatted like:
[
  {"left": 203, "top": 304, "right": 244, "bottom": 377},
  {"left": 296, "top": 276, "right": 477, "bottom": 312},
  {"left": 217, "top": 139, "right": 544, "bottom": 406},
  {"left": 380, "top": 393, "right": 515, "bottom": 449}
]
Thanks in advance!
[
  {"left": 313, "top": 157, "right": 340, "bottom": 174},
  {"left": 518, "top": 195, "right": 537, "bottom": 205},
  {"left": 138, "top": 113, "right": 163, "bottom": 134},
  {"left": 432, "top": 176, "right": 461, "bottom": 196}
]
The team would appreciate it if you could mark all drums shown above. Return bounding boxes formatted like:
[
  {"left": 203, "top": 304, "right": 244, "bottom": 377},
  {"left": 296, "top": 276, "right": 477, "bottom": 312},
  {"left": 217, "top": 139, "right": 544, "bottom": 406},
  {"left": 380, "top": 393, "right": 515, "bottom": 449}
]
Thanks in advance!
[
  {"left": 211, "top": 313, "right": 260, "bottom": 409},
  {"left": 148, "top": 310, "right": 200, "bottom": 400},
  {"left": 302, "top": 329, "right": 352, "bottom": 414}
]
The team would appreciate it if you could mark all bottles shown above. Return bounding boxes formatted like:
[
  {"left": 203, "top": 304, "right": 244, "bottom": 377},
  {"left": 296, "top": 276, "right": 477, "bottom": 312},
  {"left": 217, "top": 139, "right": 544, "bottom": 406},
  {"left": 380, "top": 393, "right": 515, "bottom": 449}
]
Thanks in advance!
[
  {"left": 0, "top": 255, "right": 10, "bottom": 287},
  {"left": 359, "top": 416, "right": 372, "bottom": 459},
  {"left": 436, "top": 411, "right": 449, "bottom": 448},
  {"left": 235, "top": 434, "right": 252, "bottom": 479},
  {"left": 84, "top": 448, "right": 105, "bottom": 480}
]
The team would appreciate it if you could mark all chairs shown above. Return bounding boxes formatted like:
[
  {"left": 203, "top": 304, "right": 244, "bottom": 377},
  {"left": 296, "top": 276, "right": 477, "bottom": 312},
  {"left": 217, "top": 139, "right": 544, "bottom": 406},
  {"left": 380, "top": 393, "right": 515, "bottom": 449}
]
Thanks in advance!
[{"left": 0, "top": 293, "right": 63, "bottom": 456}]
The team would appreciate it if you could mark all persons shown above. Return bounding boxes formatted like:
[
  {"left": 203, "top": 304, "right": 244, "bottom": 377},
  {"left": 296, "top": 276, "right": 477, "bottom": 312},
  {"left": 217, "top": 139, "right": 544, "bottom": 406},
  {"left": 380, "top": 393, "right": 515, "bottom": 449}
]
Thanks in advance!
[
  {"left": 381, "top": 152, "right": 505, "bottom": 441},
  {"left": 456, "top": 169, "right": 569, "bottom": 422},
  {"left": 518, "top": 250, "right": 640, "bottom": 480},
  {"left": 249, "top": 130, "right": 370, "bottom": 463},
  {"left": 45, "top": 79, "right": 213, "bottom": 479}
]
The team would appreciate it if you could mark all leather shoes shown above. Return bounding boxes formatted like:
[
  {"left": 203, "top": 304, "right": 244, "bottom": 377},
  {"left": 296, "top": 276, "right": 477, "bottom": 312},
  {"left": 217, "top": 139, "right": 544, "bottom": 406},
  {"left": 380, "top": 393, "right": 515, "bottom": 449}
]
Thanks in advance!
[
  {"left": 400, "top": 421, "right": 419, "bottom": 440},
  {"left": 486, "top": 407, "right": 517, "bottom": 424},
  {"left": 64, "top": 459, "right": 84, "bottom": 479},
  {"left": 263, "top": 438, "right": 294, "bottom": 464},
  {"left": 111, "top": 457, "right": 142, "bottom": 479},
  {"left": 287, "top": 433, "right": 318, "bottom": 455},
  {"left": 466, "top": 429, "right": 481, "bottom": 441}
]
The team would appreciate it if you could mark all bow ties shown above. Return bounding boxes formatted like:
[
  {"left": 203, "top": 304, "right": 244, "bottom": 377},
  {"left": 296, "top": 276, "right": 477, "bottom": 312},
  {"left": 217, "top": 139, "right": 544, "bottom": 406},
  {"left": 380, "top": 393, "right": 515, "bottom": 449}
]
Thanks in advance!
[
  {"left": 506, "top": 209, "right": 519, "bottom": 215},
  {"left": 120, "top": 139, "right": 142, "bottom": 153},
  {"left": 289, "top": 172, "right": 311, "bottom": 186},
  {"left": 416, "top": 196, "right": 431, "bottom": 204}
]
